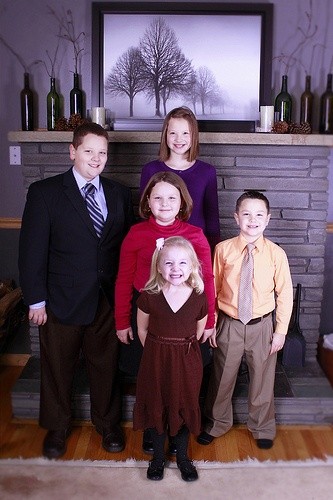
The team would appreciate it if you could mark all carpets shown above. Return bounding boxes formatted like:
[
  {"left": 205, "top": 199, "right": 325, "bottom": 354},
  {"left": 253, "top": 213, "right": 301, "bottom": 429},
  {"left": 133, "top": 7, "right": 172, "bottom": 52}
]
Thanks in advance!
[{"left": 0, "top": 457, "right": 333, "bottom": 500}]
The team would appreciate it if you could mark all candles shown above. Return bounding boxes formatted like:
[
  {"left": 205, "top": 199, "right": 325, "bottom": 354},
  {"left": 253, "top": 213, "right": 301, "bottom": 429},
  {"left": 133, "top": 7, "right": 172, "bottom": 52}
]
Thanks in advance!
[
  {"left": 92, "top": 106, "right": 105, "bottom": 125},
  {"left": 261, "top": 106, "right": 274, "bottom": 130}
]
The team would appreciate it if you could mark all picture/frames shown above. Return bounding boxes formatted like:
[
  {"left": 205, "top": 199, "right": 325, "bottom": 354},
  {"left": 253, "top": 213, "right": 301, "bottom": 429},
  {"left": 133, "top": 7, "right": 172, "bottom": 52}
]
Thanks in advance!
[{"left": 92, "top": 1, "right": 274, "bottom": 135}]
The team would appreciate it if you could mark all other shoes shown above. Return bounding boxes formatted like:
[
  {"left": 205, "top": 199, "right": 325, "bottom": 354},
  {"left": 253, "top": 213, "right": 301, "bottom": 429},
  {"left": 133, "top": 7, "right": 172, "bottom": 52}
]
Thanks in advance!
[
  {"left": 257, "top": 438, "right": 273, "bottom": 449},
  {"left": 196, "top": 431, "right": 214, "bottom": 445}
]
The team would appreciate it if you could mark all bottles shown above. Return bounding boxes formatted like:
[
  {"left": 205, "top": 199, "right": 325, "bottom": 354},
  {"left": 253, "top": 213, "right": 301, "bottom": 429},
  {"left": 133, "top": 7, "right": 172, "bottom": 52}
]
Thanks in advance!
[
  {"left": 21, "top": 72, "right": 33, "bottom": 132},
  {"left": 70, "top": 73, "right": 83, "bottom": 118},
  {"left": 274, "top": 76, "right": 292, "bottom": 124},
  {"left": 300, "top": 75, "right": 314, "bottom": 128},
  {"left": 47, "top": 78, "right": 59, "bottom": 130},
  {"left": 319, "top": 73, "right": 333, "bottom": 134}
]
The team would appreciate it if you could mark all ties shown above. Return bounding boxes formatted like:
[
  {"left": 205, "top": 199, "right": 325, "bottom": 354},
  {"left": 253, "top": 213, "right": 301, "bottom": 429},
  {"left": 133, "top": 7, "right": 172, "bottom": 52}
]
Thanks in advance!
[
  {"left": 238, "top": 244, "right": 256, "bottom": 325},
  {"left": 81, "top": 184, "right": 105, "bottom": 239}
]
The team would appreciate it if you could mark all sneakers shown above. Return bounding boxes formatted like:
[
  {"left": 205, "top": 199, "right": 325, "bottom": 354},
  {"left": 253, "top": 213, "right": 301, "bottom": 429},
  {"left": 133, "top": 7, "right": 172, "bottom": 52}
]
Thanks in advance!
[
  {"left": 146, "top": 454, "right": 166, "bottom": 481},
  {"left": 177, "top": 454, "right": 199, "bottom": 481}
]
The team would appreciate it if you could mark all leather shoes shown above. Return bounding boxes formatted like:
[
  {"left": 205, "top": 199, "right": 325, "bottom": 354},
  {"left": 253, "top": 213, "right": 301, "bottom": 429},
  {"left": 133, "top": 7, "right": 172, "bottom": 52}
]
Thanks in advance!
[
  {"left": 96, "top": 424, "right": 124, "bottom": 452},
  {"left": 142, "top": 429, "right": 157, "bottom": 454},
  {"left": 168, "top": 429, "right": 177, "bottom": 455},
  {"left": 44, "top": 427, "right": 72, "bottom": 458}
]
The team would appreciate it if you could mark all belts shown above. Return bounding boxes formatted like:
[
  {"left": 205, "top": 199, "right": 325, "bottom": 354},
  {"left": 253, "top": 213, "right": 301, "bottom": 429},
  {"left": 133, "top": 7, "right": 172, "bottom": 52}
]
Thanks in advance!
[{"left": 225, "top": 314, "right": 270, "bottom": 325}]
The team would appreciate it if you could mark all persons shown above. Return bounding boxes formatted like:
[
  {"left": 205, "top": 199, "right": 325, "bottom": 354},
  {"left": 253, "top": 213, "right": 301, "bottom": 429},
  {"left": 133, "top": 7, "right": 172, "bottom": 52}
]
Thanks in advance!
[
  {"left": 140, "top": 106, "right": 220, "bottom": 265},
  {"left": 115, "top": 172, "right": 217, "bottom": 482},
  {"left": 198, "top": 191, "right": 293, "bottom": 450},
  {"left": 18, "top": 124, "right": 137, "bottom": 460}
]
